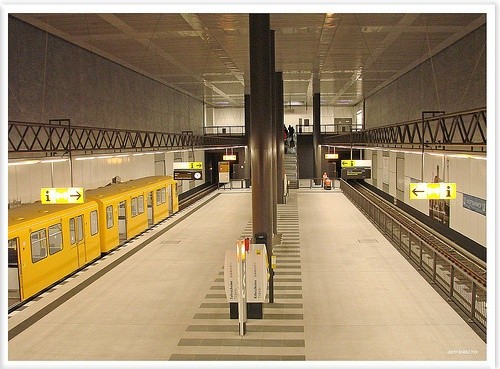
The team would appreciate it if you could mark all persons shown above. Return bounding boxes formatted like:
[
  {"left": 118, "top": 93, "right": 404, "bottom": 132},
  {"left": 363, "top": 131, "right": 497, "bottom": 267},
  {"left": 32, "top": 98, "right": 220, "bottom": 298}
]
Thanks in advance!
[
  {"left": 323, "top": 171, "right": 327, "bottom": 181},
  {"left": 284, "top": 124, "right": 296, "bottom": 154}
]
